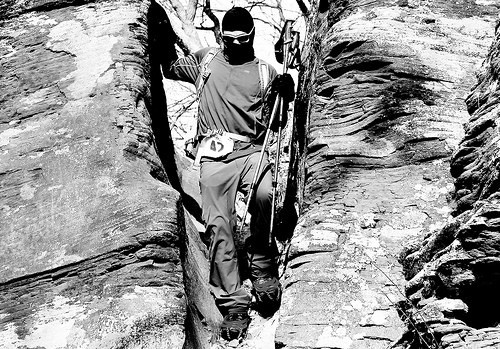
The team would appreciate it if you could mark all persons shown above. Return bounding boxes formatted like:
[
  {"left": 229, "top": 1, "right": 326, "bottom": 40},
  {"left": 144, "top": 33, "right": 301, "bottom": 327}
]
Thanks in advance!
[{"left": 156, "top": 6, "right": 296, "bottom": 341}]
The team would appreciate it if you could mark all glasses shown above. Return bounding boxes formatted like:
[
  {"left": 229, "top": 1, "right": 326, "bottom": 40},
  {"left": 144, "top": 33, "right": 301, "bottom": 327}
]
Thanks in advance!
[{"left": 221, "top": 26, "right": 254, "bottom": 44}]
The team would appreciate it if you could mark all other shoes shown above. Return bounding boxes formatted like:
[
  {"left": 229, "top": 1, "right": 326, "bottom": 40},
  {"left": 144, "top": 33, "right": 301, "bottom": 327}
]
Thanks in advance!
[
  {"left": 248, "top": 262, "right": 279, "bottom": 303},
  {"left": 219, "top": 306, "right": 247, "bottom": 336}
]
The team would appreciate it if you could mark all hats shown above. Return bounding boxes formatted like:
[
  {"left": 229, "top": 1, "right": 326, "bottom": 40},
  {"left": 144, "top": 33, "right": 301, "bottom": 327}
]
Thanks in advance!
[{"left": 221, "top": 7, "right": 256, "bottom": 65}]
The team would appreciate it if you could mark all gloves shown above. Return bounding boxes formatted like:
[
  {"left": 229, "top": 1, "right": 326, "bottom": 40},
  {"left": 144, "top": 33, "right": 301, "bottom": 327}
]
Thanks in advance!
[
  {"left": 267, "top": 73, "right": 295, "bottom": 112},
  {"left": 150, "top": 23, "right": 177, "bottom": 63}
]
